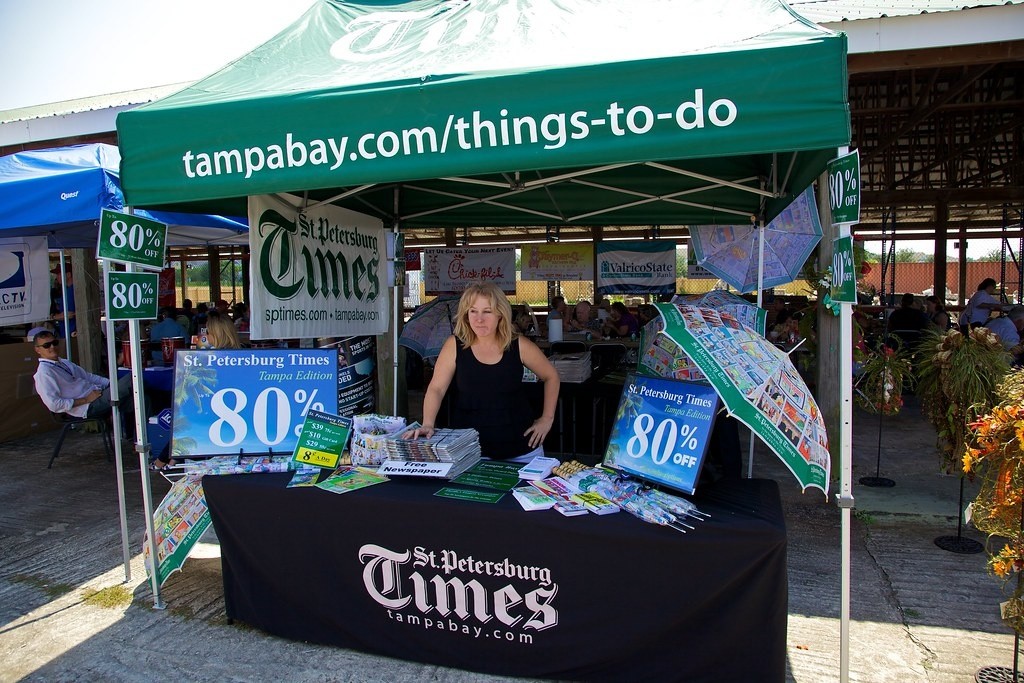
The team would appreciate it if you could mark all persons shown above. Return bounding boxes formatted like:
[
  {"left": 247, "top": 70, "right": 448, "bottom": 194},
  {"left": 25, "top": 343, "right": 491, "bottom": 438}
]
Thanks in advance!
[
  {"left": 400, "top": 281, "right": 560, "bottom": 465},
  {"left": 32, "top": 331, "right": 153, "bottom": 454},
  {"left": 148, "top": 311, "right": 242, "bottom": 470},
  {"left": 108, "top": 277, "right": 1024, "bottom": 365}
]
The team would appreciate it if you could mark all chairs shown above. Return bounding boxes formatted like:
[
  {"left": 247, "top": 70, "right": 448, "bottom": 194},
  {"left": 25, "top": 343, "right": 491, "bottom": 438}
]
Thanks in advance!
[
  {"left": 550, "top": 340, "right": 587, "bottom": 355},
  {"left": 32, "top": 382, "right": 116, "bottom": 470},
  {"left": 890, "top": 330, "right": 922, "bottom": 348},
  {"left": 589, "top": 342, "right": 628, "bottom": 353}
]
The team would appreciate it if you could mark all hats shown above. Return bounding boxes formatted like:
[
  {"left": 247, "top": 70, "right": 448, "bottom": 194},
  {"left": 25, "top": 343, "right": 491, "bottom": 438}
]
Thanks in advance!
[{"left": 50, "top": 262, "right": 72, "bottom": 274}]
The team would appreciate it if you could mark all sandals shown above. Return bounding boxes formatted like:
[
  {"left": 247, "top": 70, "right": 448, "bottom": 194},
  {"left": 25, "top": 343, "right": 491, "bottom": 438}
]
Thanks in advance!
[{"left": 150, "top": 458, "right": 181, "bottom": 472}]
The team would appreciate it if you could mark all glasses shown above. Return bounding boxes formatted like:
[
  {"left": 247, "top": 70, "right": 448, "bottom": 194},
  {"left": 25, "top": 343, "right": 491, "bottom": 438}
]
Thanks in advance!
[{"left": 34, "top": 340, "right": 59, "bottom": 348}]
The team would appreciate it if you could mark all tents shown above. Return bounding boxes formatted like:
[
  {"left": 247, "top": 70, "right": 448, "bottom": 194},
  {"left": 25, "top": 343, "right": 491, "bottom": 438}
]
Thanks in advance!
[
  {"left": 0, "top": 142, "right": 249, "bottom": 587},
  {"left": 113, "top": 0, "right": 860, "bottom": 682}
]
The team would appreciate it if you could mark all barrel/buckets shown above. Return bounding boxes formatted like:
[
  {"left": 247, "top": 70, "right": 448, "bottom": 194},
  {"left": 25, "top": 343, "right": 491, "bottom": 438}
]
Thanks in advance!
[
  {"left": 122, "top": 338, "right": 149, "bottom": 369},
  {"left": 161, "top": 336, "right": 186, "bottom": 364}
]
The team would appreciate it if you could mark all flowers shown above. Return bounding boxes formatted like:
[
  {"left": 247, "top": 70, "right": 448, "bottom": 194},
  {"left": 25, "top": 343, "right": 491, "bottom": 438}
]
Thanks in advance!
[
  {"left": 960, "top": 365, "right": 1024, "bottom": 638},
  {"left": 851, "top": 234, "right": 872, "bottom": 280},
  {"left": 914, "top": 324, "right": 1013, "bottom": 480},
  {"left": 853, "top": 334, "right": 916, "bottom": 417},
  {"left": 818, "top": 266, "right": 840, "bottom": 316}
]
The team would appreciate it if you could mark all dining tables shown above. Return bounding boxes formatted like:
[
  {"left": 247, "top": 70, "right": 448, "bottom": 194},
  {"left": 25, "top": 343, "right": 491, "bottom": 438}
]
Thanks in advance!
[{"left": 117, "top": 361, "right": 174, "bottom": 392}]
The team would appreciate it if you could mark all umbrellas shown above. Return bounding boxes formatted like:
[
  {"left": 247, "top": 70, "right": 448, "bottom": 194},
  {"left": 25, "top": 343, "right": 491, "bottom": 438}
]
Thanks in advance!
[
  {"left": 550, "top": 459, "right": 712, "bottom": 536},
  {"left": 168, "top": 452, "right": 353, "bottom": 478},
  {"left": 400, "top": 294, "right": 469, "bottom": 361},
  {"left": 649, "top": 302, "right": 831, "bottom": 508},
  {"left": 688, "top": 183, "right": 828, "bottom": 293},
  {"left": 636, "top": 290, "right": 769, "bottom": 387},
  {"left": 139, "top": 470, "right": 216, "bottom": 593}
]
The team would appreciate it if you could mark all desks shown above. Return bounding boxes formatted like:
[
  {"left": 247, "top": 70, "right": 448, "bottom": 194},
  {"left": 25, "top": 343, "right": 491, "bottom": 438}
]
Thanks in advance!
[
  {"left": 522, "top": 328, "right": 641, "bottom": 460},
  {"left": 199, "top": 459, "right": 788, "bottom": 682}
]
